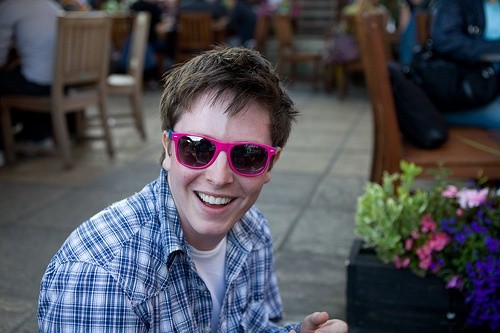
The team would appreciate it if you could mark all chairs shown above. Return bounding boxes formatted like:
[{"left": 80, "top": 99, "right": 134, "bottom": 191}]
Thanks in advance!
[
  {"left": 0, "top": 11, "right": 116, "bottom": 170},
  {"left": 82, "top": 10, "right": 428, "bottom": 142},
  {"left": 353, "top": 11, "right": 498, "bottom": 192}
]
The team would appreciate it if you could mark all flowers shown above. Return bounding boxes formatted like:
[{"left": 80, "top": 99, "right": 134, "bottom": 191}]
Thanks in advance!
[{"left": 353, "top": 160, "right": 499, "bottom": 333}]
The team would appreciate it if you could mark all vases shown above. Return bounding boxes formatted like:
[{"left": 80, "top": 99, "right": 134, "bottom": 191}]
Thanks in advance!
[{"left": 344, "top": 236, "right": 488, "bottom": 332}]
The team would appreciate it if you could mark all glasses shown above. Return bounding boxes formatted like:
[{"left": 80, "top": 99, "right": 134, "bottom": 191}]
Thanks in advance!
[{"left": 168, "top": 127, "right": 281, "bottom": 177}]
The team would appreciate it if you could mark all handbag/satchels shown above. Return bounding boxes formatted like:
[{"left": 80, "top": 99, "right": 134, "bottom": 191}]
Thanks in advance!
[
  {"left": 388, "top": 62, "right": 449, "bottom": 151},
  {"left": 411, "top": 0, "right": 500, "bottom": 115}
]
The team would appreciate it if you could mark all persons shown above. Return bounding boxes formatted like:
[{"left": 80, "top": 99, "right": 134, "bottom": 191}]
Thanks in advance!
[
  {"left": 0, "top": 1, "right": 68, "bottom": 160},
  {"left": 92, "top": 1, "right": 260, "bottom": 94},
  {"left": 408, "top": 1, "right": 500, "bottom": 131},
  {"left": 331, "top": 1, "right": 434, "bottom": 92},
  {"left": 36, "top": 46, "right": 351, "bottom": 333}
]
